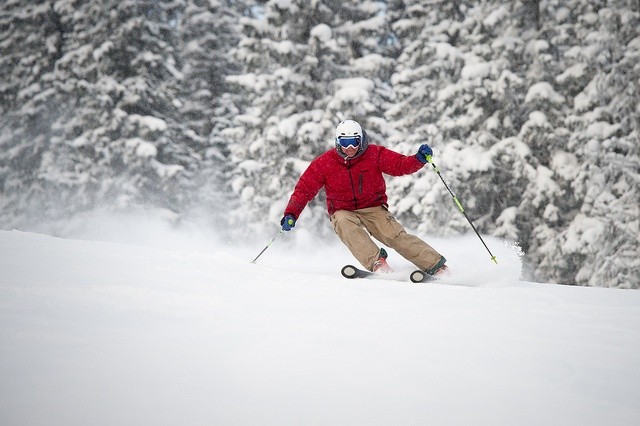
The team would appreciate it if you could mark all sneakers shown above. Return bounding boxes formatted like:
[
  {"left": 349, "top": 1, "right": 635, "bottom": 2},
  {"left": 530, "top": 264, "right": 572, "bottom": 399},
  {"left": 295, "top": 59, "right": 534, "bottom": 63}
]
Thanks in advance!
[
  {"left": 371, "top": 257, "right": 393, "bottom": 273},
  {"left": 434, "top": 265, "right": 450, "bottom": 278}
]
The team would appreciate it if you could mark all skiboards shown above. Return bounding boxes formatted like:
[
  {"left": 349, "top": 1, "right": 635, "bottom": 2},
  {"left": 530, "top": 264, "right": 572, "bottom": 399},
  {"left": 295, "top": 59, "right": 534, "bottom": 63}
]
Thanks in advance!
[{"left": 341, "top": 265, "right": 440, "bottom": 284}]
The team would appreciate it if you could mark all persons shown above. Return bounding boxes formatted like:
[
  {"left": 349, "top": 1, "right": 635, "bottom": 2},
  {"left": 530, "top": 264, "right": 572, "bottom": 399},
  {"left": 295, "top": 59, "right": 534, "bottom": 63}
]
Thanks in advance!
[{"left": 280, "top": 119, "right": 453, "bottom": 278}]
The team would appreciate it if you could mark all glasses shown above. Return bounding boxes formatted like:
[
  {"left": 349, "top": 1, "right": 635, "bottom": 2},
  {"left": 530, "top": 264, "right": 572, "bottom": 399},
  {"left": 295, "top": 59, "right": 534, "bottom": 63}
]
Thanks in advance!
[{"left": 337, "top": 138, "right": 361, "bottom": 149}]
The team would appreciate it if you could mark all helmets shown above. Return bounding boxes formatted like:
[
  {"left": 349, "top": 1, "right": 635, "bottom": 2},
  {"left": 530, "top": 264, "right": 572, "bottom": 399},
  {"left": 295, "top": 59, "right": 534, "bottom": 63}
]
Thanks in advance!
[{"left": 335, "top": 120, "right": 363, "bottom": 149}]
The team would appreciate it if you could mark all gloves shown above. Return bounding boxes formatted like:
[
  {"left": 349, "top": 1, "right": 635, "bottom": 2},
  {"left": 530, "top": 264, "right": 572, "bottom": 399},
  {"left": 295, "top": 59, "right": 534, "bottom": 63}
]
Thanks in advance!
[
  {"left": 417, "top": 144, "right": 433, "bottom": 164},
  {"left": 281, "top": 216, "right": 295, "bottom": 231}
]
